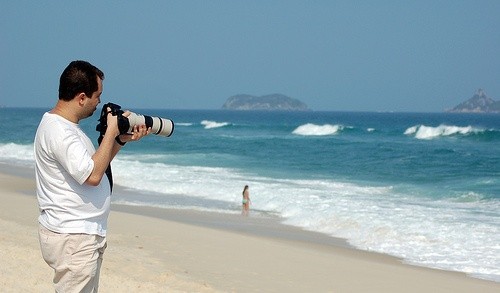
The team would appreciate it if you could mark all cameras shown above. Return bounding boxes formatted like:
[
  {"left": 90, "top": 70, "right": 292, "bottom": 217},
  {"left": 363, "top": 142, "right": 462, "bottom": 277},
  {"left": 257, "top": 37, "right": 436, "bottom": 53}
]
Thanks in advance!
[{"left": 96, "top": 103, "right": 174, "bottom": 137}]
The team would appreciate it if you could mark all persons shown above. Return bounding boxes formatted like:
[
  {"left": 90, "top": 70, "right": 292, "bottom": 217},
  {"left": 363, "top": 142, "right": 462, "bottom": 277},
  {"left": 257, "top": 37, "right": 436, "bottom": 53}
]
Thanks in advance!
[
  {"left": 242, "top": 185, "right": 251, "bottom": 218},
  {"left": 33, "top": 60, "right": 152, "bottom": 293}
]
